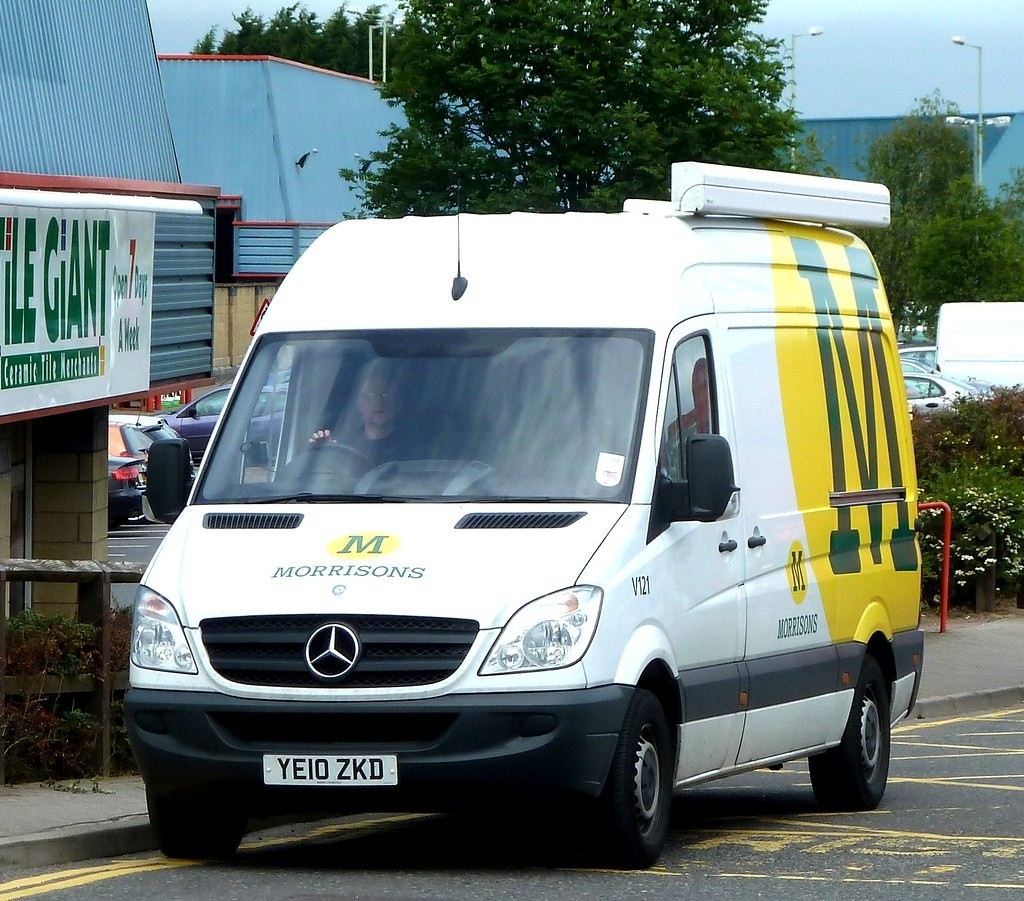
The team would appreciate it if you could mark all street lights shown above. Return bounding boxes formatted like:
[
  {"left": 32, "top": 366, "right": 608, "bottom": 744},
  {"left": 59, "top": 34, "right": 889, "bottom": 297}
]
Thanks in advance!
[
  {"left": 946, "top": 115, "right": 1012, "bottom": 190},
  {"left": 368, "top": 22, "right": 407, "bottom": 82},
  {"left": 789, "top": 26, "right": 825, "bottom": 174},
  {"left": 945, "top": 37, "right": 985, "bottom": 196},
  {"left": 346, "top": 9, "right": 387, "bottom": 83}
]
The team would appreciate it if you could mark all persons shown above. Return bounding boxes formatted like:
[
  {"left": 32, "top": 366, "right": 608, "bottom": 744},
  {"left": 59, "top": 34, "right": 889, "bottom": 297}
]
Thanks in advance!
[
  {"left": 667, "top": 358, "right": 710, "bottom": 481},
  {"left": 309, "top": 358, "right": 425, "bottom": 468}
]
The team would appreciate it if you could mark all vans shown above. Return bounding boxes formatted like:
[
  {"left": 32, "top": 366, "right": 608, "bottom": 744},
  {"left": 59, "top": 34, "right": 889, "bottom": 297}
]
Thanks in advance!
[{"left": 123, "top": 158, "right": 927, "bottom": 870}]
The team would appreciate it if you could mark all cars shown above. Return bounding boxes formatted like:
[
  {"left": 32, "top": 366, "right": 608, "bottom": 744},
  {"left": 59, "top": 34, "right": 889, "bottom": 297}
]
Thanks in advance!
[
  {"left": 153, "top": 380, "right": 288, "bottom": 468},
  {"left": 107, "top": 452, "right": 149, "bottom": 530},
  {"left": 897, "top": 339, "right": 1010, "bottom": 423}
]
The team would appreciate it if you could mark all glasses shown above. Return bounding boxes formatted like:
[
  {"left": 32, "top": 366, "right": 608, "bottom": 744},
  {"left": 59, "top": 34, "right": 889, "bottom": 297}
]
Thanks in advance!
[{"left": 361, "top": 391, "right": 398, "bottom": 401}]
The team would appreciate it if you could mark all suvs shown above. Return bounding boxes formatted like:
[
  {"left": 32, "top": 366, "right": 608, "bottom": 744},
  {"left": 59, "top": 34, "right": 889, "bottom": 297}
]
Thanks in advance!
[{"left": 108, "top": 417, "right": 197, "bottom": 490}]
